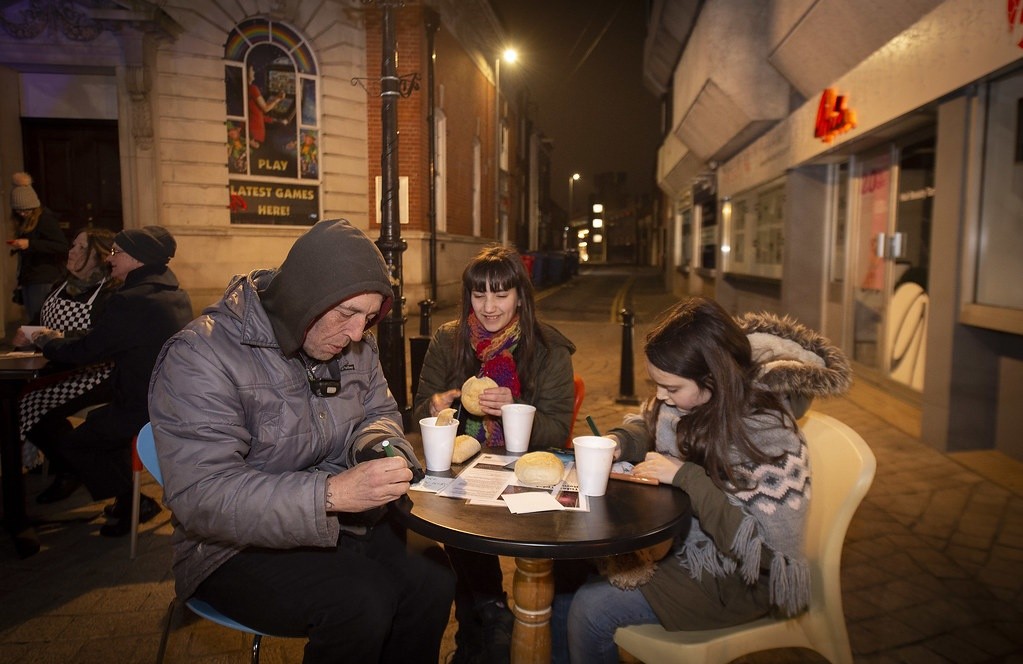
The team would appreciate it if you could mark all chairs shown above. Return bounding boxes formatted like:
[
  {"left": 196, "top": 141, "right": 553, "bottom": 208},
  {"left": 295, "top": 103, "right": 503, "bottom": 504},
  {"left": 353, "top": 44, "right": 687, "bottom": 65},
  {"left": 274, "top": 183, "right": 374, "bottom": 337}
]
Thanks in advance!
[
  {"left": 137, "top": 423, "right": 271, "bottom": 664},
  {"left": 614, "top": 408, "right": 876, "bottom": 664}
]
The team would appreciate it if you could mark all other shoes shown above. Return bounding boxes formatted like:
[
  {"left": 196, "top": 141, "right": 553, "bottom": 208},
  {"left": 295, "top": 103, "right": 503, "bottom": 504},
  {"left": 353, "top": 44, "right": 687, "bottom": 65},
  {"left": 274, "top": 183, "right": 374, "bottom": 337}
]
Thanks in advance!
[
  {"left": 100, "top": 495, "right": 162, "bottom": 536},
  {"left": 446, "top": 591, "right": 514, "bottom": 664},
  {"left": 37, "top": 476, "right": 80, "bottom": 505},
  {"left": 0, "top": 535, "right": 42, "bottom": 562}
]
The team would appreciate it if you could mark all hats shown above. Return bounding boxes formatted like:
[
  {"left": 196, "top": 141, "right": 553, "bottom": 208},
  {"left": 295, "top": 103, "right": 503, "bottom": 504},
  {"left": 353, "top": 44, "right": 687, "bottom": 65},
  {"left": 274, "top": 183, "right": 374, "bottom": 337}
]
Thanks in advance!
[
  {"left": 114, "top": 226, "right": 177, "bottom": 264},
  {"left": 10, "top": 172, "right": 41, "bottom": 210}
]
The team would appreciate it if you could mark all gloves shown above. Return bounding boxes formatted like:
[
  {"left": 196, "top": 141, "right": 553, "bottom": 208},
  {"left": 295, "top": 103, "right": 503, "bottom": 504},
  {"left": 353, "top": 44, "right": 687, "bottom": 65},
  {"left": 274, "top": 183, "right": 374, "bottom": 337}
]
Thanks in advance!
[{"left": 357, "top": 435, "right": 426, "bottom": 485}]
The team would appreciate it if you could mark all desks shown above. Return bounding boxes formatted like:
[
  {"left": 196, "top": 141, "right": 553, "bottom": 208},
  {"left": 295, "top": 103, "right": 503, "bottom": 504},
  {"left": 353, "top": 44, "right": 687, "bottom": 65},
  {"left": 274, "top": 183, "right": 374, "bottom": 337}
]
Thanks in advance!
[
  {"left": 0, "top": 350, "right": 51, "bottom": 556},
  {"left": 387, "top": 442, "right": 693, "bottom": 664}
]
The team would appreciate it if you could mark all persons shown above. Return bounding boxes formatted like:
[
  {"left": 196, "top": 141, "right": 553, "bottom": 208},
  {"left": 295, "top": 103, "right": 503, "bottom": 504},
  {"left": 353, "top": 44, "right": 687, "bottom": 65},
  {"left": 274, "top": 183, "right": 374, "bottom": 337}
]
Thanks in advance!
[
  {"left": 552, "top": 296, "right": 853, "bottom": 664},
  {"left": 147, "top": 218, "right": 455, "bottom": 664},
  {"left": 11, "top": 228, "right": 114, "bottom": 491},
  {"left": 37, "top": 226, "right": 194, "bottom": 537},
  {"left": 411, "top": 246, "right": 578, "bottom": 664},
  {"left": 9, "top": 173, "right": 70, "bottom": 326}
]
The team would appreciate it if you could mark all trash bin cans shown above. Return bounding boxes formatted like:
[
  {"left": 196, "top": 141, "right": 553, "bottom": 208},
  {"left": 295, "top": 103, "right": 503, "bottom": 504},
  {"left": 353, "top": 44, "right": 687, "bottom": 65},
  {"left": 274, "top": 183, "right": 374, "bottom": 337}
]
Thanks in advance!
[{"left": 522, "top": 249, "right": 579, "bottom": 289}]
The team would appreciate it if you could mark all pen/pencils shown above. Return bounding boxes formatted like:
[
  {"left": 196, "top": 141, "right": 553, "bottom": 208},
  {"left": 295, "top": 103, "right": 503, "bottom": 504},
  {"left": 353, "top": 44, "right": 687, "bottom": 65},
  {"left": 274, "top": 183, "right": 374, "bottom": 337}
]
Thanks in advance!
[{"left": 586, "top": 415, "right": 600, "bottom": 436}]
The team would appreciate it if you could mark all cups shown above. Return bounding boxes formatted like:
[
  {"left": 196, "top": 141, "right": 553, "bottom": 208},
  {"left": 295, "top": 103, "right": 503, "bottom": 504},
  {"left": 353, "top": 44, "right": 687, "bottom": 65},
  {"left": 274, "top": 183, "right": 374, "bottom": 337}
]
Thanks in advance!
[
  {"left": 572, "top": 435, "right": 618, "bottom": 496},
  {"left": 419, "top": 417, "right": 460, "bottom": 472},
  {"left": 501, "top": 403, "right": 536, "bottom": 452}
]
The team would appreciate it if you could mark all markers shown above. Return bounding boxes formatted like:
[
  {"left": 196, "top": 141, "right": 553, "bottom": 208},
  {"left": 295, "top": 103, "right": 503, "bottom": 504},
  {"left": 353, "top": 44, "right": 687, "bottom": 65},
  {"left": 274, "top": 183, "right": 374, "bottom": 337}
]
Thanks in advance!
[{"left": 382, "top": 441, "right": 395, "bottom": 456}]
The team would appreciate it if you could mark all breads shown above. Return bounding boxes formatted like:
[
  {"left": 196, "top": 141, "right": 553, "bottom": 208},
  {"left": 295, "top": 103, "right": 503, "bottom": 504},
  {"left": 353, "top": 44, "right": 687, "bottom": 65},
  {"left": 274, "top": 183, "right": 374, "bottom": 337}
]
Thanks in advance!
[
  {"left": 514, "top": 451, "right": 565, "bottom": 486},
  {"left": 460, "top": 376, "right": 499, "bottom": 416},
  {"left": 451, "top": 434, "right": 481, "bottom": 463}
]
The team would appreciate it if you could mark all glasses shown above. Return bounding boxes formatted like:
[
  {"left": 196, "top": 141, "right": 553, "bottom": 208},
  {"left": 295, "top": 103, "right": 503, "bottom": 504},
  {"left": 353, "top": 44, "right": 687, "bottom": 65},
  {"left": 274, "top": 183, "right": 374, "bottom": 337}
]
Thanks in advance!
[{"left": 109, "top": 247, "right": 126, "bottom": 257}]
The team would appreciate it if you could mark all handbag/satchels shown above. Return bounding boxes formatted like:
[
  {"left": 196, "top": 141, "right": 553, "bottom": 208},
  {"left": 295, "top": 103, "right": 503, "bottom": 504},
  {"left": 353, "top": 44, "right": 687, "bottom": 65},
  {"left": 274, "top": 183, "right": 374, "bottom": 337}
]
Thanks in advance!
[{"left": 11, "top": 288, "right": 23, "bottom": 305}]
